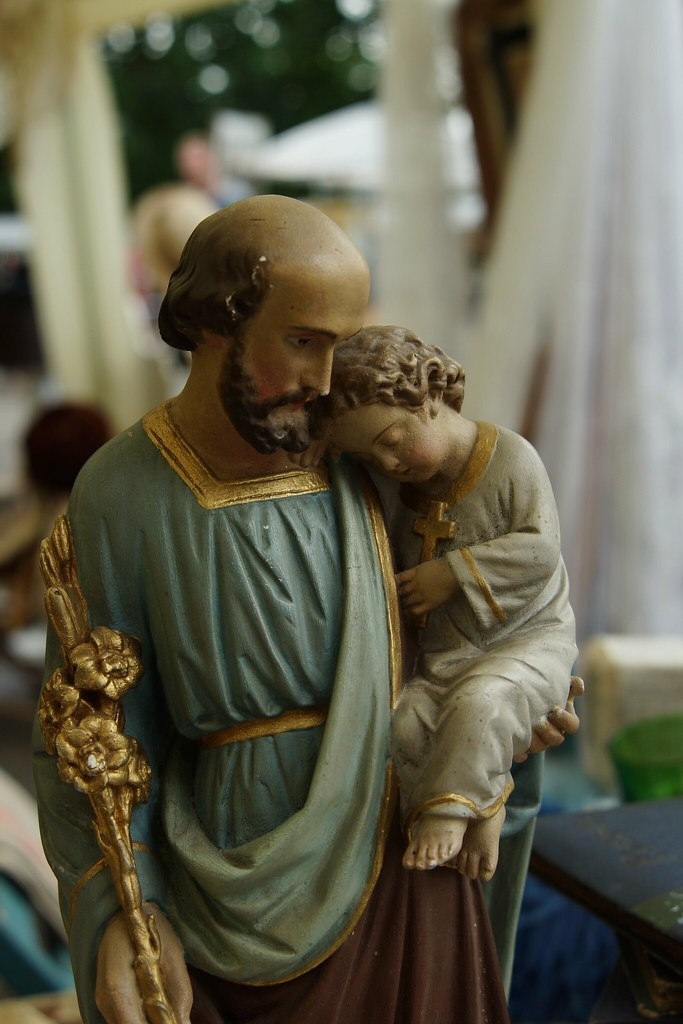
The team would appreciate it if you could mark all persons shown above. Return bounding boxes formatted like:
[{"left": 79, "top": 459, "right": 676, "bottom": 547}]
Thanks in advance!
[
  {"left": 321, "top": 319, "right": 576, "bottom": 885},
  {"left": 33, "top": 190, "right": 584, "bottom": 1021}
]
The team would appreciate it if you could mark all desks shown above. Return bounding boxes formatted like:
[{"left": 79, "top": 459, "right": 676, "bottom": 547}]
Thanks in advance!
[{"left": 528, "top": 795, "right": 683, "bottom": 1024}]
